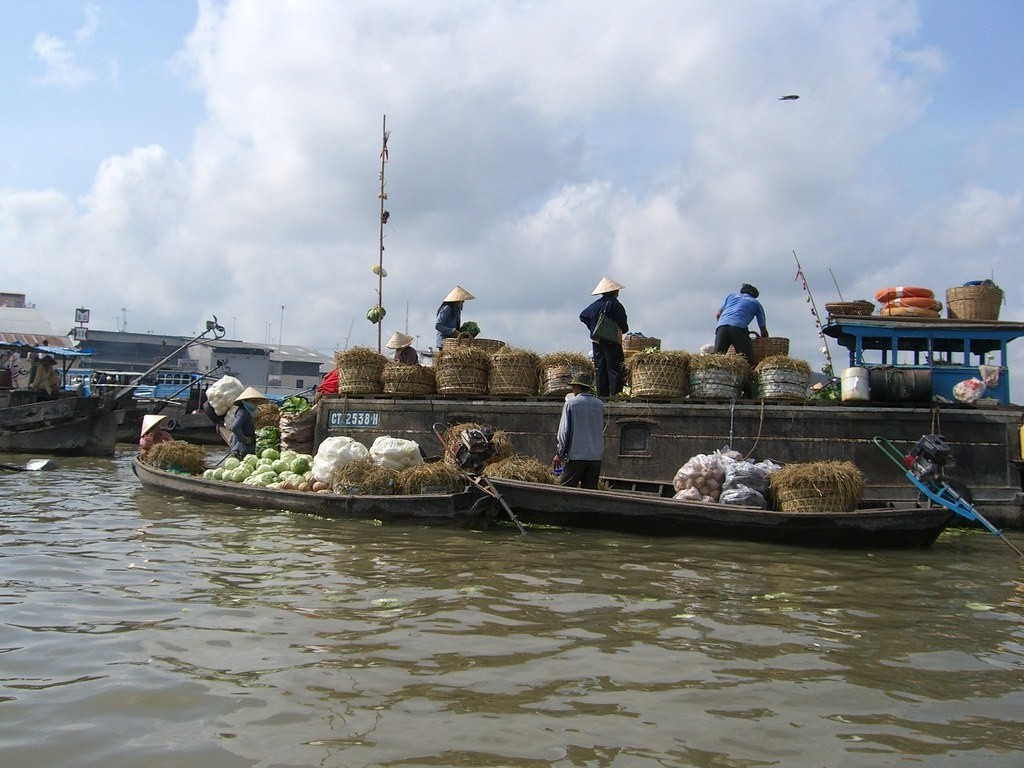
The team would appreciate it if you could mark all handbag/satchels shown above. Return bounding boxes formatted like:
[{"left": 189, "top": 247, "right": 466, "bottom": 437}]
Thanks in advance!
[{"left": 592, "top": 296, "right": 623, "bottom": 347}]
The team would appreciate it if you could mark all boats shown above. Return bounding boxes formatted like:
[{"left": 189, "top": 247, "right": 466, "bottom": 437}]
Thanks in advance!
[
  {"left": 0, "top": 341, "right": 119, "bottom": 457},
  {"left": 461, "top": 435, "right": 958, "bottom": 550},
  {"left": 312, "top": 314, "right": 1024, "bottom": 526},
  {"left": 131, "top": 452, "right": 498, "bottom": 523},
  {"left": 54, "top": 365, "right": 285, "bottom": 445}
]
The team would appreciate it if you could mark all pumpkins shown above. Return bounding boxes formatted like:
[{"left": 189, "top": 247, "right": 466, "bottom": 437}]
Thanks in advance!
[{"left": 277, "top": 473, "right": 330, "bottom": 494}]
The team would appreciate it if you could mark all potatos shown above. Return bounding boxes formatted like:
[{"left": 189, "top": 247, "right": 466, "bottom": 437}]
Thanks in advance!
[{"left": 674, "top": 454, "right": 726, "bottom": 501}]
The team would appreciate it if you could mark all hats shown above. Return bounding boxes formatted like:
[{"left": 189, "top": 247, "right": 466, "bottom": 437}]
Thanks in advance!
[
  {"left": 232, "top": 387, "right": 269, "bottom": 406},
  {"left": 384, "top": 331, "right": 416, "bottom": 349},
  {"left": 39, "top": 355, "right": 57, "bottom": 366},
  {"left": 567, "top": 372, "right": 591, "bottom": 388},
  {"left": 442, "top": 286, "right": 476, "bottom": 302},
  {"left": 140, "top": 414, "right": 168, "bottom": 437},
  {"left": 590, "top": 275, "right": 626, "bottom": 295}
]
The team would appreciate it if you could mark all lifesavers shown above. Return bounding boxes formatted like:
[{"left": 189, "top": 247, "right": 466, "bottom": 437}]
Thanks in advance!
[
  {"left": 166, "top": 417, "right": 178, "bottom": 432},
  {"left": 918, "top": 475, "right": 970, "bottom": 528}
]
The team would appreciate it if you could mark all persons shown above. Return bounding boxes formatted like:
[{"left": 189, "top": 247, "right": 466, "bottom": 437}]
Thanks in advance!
[
  {"left": 579, "top": 277, "right": 629, "bottom": 399},
  {"left": 435, "top": 286, "right": 475, "bottom": 350},
  {"left": 8, "top": 338, "right": 62, "bottom": 398},
  {"left": 713, "top": 283, "right": 769, "bottom": 368},
  {"left": 552, "top": 371, "right": 606, "bottom": 490},
  {"left": 228, "top": 386, "right": 268, "bottom": 462},
  {"left": 384, "top": 331, "right": 419, "bottom": 366},
  {"left": 140, "top": 415, "right": 173, "bottom": 459}
]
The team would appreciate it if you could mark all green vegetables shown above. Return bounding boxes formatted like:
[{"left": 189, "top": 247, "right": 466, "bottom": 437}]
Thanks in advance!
[
  {"left": 278, "top": 396, "right": 311, "bottom": 416},
  {"left": 255, "top": 426, "right": 281, "bottom": 457},
  {"left": 808, "top": 386, "right": 841, "bottom": 400}
]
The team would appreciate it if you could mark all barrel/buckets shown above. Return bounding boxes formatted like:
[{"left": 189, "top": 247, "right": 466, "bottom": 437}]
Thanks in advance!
[
  {"left": 870, "top": 369, "right": 932, "bottom": 404},
  {"left": 841, "top": 367, "right": 870, "bottom": 402}
]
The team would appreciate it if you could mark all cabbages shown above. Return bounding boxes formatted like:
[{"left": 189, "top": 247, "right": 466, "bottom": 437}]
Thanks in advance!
[
  {"left": 366, "top": 307, "right": 385, "bottom": 323},
  {"left": 201, "top": 448, "right": 313, "bottom": 489},
  {"left": 372, "top": 265, "right": 387, "bottom": 277},
  {"left": 459, "top": 321, "right": 481, "bottom": 339}
]
